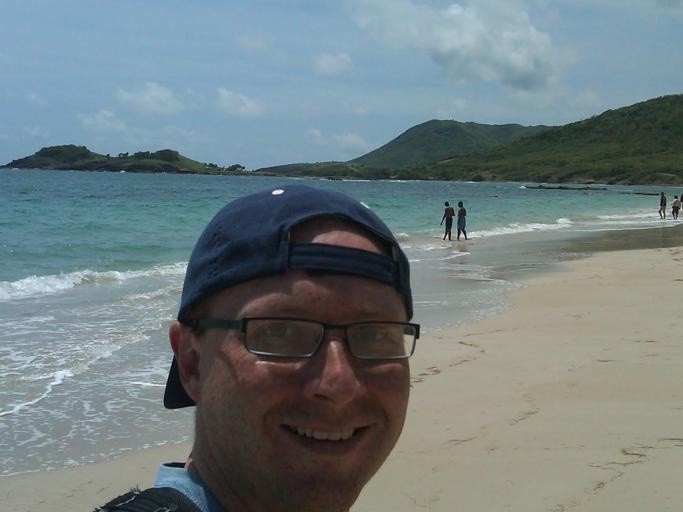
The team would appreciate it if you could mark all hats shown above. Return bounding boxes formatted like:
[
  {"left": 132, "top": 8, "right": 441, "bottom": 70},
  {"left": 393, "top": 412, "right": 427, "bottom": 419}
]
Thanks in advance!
[{"left": 163, "top": 184, "right": 413, "bottom": 407}]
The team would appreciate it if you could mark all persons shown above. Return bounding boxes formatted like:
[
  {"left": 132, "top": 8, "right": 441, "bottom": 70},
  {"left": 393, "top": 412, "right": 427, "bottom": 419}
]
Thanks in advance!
[
  {"left": 438, "top": 201, "right": 455, "bottom": 241},
  {"left": 658, "top": 191, "right": 667, "bottom": 219},
  {"left": 454, "top": 201, "right": 468, "bottom": 240},
  {"left": 88, "top": 183, "right": 422, "bottom": 512},
  {"left": 670, "top": 195, "right": 681, "bottom": 220}
]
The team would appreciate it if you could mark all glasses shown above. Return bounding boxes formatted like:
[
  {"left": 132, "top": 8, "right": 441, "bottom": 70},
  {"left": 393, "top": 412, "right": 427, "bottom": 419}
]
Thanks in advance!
[{"left": 186, "top": 316, "right": 420, "bottom": 361}]
